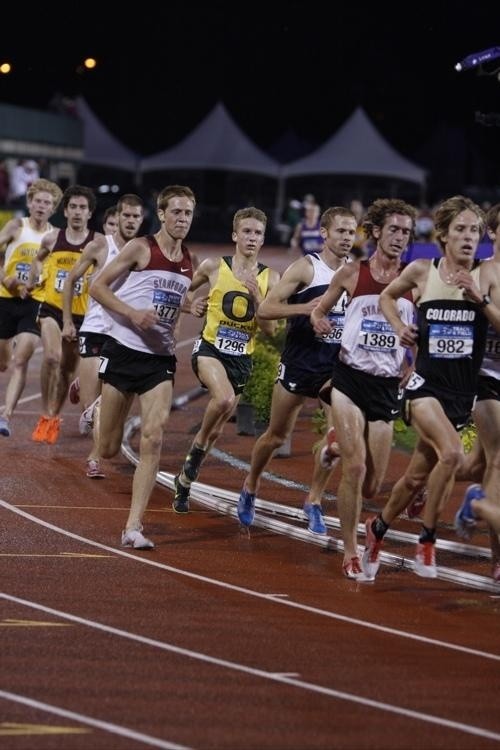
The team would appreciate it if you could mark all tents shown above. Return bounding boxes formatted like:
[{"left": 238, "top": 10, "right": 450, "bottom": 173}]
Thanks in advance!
[
  {"left": 136, "top": 104, "right": 287, "bottom": 227},
  {"left": 74, "top": 95, "right": 137, "bottom": 172},
  {"left": 285, "top": 107, "right": 427, "bottom": 208}
]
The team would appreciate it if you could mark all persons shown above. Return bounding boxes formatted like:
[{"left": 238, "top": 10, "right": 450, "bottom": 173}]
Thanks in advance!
[
  {"left": 236, "top": 205, "right": 358, "bottom": 537},
  {"left": 455, "top": 202, "right": 499, "bottom": 581},
  {"left": 401, "top": 218, "right": 444, "bottom": 263},
  {"left": 103, "top": 206, "right": 120, "bottom": 235},
  {"left": 347, "top": 198, "right": 372, "bottom": 248},
  {"left": 88, "top": 185, "right": 211, "bottom": 550},
  {"left": 0, "top": 179, "right": 63, "bottom": 438},
  {"left": 18, "top": 184, "right": 103, "bottom": 446},
  {"left": 172, "top": 207, "right": 280, "bottom": 515},
  {"left": 475, "top": 199, "right": 494, "bottom": 261},
  {"left": 310, "top": 199, "right": 418, "bottom": 580},
  {"left": 291, "top": 197, "right": 325, "bottom": 255},
  {"left": 60, "top": 197, "right": 147, "bottom": 480},
  {"left": 360, "top": 195, "right": 499, "bottom": 581},
  {"left": 10, "top": 156, "right": 39, "bottom": 198}
]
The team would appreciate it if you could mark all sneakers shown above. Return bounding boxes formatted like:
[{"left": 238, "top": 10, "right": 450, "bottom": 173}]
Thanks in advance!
[
  {"left": 320, "top": 426, "right": 339, "bottom": 469},
  {"left": 405, "top": 485, "right": 428, "bottom": 519},
  {"left": 454, "top": 483, "right": 484, "bottom": 540},
  {"left": 84, "top": 459, "right": 106, "bottom": 479},
  {"left": 363, "top": 517, "right": 382, "bottom": 577},
  {"left": 69, "top": 376, "right": 81, "bottom": 404},
  {"left": 171, "top": 475, "right": 191, "bottom": 514},
  {"left": 78, "top": 394, "right": 101, "bottom": 436},
  {"left": 0, "top": 416, "right": 11, "bottom": 437},
  {"left": 342, "top": 557, "right": 364, "bottom": 578},
  {"left": 184, "top": 449, "right": 200, "bottom": 482},
  {"left": 47, "top": 417, "right": 61, "bottom": 444},
  {"left": 238, "top": 489, "right": 255, "bottom": 526},
  {"left": 304, "top": 503, "right": 327, "bottom": 535},
  {"left": 120, "top": 530, "right": 154, "bottom": 550},
  {"left": 414, "top": 543, "right": 437, "bottom": 578},
  {"left": 31, "top": 416, "right": 49, "bottom": 441}
]
